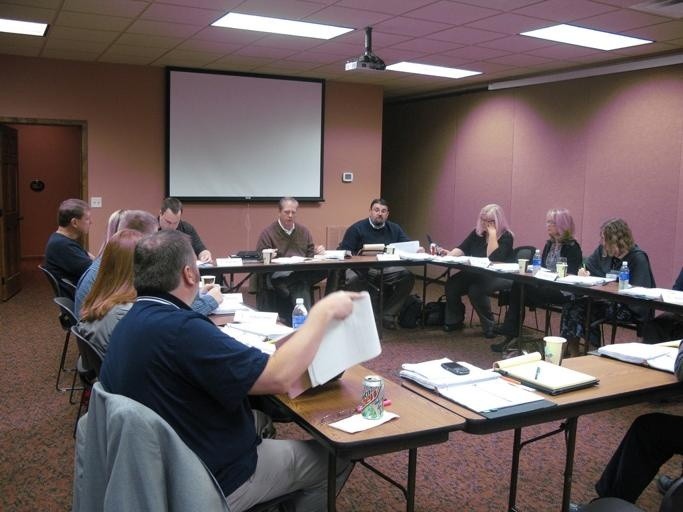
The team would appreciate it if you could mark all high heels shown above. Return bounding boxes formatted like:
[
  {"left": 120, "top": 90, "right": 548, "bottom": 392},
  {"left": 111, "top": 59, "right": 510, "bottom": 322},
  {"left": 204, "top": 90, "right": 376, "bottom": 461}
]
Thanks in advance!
[{"left": 561, "top": 339, "right": 579, "bottom": 358}]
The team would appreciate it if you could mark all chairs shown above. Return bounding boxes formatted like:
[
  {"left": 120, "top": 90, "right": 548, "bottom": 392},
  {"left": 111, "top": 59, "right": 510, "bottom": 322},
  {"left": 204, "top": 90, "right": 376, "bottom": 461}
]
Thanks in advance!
[
  {"left": 68, "top": 325, "right": 109, "bottom": 438},
  {"left": 326, "top": 226, "right": 350, "bottom": 248},
  {"left": 59, "top": 274, "right": 80, "bottom": 301},
  {"left": 51, "top": 295, "right": 103, "bottom": 391},
  {"left": 470, "top": 245, "right": 536, "bottom": 335},
  {"left": 39, "top": 263, "right": 86, "bottom": 371}
]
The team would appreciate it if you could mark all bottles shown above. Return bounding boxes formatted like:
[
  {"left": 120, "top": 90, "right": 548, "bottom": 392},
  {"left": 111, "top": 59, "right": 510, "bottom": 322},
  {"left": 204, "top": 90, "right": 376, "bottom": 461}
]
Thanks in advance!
[
  {"left": 532, "top": 250, "right": 541, "bottom": 272},
  {"left": 618, "top": 261, "right": 629, "bottom": 290},
  {"left": 361, "top": 375, "right": 384, "bottom": 420},
  {"left": 292, "top": 298, "right": 308, "bottom": 329}
]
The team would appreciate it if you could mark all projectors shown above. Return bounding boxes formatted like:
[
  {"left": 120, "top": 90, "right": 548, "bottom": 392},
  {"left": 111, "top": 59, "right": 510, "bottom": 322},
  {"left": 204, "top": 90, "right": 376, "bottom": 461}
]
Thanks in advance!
[{"left": 345, "top": 51, "right": 386, "bottom": 74}]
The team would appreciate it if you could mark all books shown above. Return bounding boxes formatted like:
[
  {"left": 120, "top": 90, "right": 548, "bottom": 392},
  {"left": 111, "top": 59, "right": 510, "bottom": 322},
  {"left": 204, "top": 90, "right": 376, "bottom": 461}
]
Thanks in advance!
[
  {"left": 314, "top": 249, "right": 352, "bottom": 261},
  {"left": 270, "top": 255, "right": 311, "bottom": 264},
  {"left": 273, "top": 290, "right": 382, "bottom": 400},
  {"left": 492, "top": 351, "right": 599, "bottom": 394},
  {"left": 596, "top": 341, "right": 677, "bottom": 373}
]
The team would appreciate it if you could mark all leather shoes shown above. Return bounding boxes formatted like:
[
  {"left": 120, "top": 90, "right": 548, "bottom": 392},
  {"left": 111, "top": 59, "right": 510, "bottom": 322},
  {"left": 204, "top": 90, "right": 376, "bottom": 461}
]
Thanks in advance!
[
  {"left": 486, "top": 331, "right": 496, "bottom": 338},
  {"left": 568, "top": 499, "right": 578, "bottom": 510},
  {"left": 500, "top": 292, "right": 509, "bottom": 306},
  {"left": 491, "top": 337, "right": 511, "bottom": 351},
  {"left": 659, "top": 473, "right": 672, "bottom": 492},
  {"left": 384, "top": 319, "right": 394, "bottom": 328},
  {"left": 445, "top": 323, "right": 464, "bottom": 330}
]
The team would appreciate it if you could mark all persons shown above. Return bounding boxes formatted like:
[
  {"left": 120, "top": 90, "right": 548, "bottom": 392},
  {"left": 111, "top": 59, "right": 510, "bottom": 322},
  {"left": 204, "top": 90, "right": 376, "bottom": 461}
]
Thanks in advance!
[
  {"left": 44, "top": 198, "right": 97, "bottom": 298},
  {"left": 642, "top": 266, "right": 683, "bottom": 344},
  {"left": 256, "top": 197, "right": 325, "bottom": 314},
  {"left": 155, "top": 197, "right": 214, "bottom": 264},
  {"left": 436, "top": 203, "right": 518, "bottom": 339},
  {"left": 340, "top": 198, "right": 426, "bottom": 330},
  {"left": 565, "top": 341, "right": 683, "bottom": 511},
  {"left": 99, "top": 228, "right": 366, "bottom": 512},
  {"left": 74, "top": 210, "right": 224, "bottom": 317},
  {"left": 77, "top": 228, "right": 144, "bottom": 355},
  {"left": 560, "top": 219, "right": 654, "bottom": 355},
  {"left": 490, "top": 206, "right": 582, "bottom": 353}
]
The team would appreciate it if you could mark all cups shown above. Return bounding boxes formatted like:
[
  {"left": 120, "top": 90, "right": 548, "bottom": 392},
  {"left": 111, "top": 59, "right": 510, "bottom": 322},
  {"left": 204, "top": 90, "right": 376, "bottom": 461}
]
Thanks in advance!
[
  {"left": 262, "top": 252, "right": 272, "bottom": 264},
  {"left": 201, "top": 276, "right": 216, "bottom": 285},
  {"left": 543, "top": 336, "right": 567, "bottom": 366},
  {"left": 518, "top": 258, "right": 530, "bottom": 272},
  {"left": 555, "top": 263, "right": 568, "bottom": 277}
]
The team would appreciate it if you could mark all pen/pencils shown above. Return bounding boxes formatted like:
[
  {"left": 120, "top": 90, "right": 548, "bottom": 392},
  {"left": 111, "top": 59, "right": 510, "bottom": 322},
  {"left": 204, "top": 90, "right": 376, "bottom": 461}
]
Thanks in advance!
[
  {"left": 502, "top": 376, "right": 521, "bottom": 385},
  {"left": 583, "top": 264, "right": 585, "bottom": 269},
  {"left": 357, "top": 400, "right": 391, "bottom": 413},
  {"left": 435, "top": 243, "right": 440, "bottom": 254},
  {"left": 535, "top": 367, "right": 540, "bottom": 379}
]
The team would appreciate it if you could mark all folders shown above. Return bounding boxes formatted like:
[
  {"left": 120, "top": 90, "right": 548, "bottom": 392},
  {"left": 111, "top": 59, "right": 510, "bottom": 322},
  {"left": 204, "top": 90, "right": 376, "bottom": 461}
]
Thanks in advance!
[{"left": 587, "top": 342, "right": 675, "bottom": 373}]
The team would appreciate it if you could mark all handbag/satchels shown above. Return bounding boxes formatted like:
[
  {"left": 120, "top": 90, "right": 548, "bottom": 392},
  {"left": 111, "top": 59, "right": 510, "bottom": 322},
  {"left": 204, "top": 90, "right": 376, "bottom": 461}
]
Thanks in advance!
[
  {"left": 425, "top": 296, "right": 466, "bottom": 327},
  {"left": 400, "top": 294, "right": 422, "bottom": 327}
]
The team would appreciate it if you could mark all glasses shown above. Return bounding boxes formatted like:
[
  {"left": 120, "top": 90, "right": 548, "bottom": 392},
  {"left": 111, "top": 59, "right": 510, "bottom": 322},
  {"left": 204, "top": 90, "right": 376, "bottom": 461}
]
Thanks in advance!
[{"left": 481, "top": 218, "right": 494, "bottom": 222}]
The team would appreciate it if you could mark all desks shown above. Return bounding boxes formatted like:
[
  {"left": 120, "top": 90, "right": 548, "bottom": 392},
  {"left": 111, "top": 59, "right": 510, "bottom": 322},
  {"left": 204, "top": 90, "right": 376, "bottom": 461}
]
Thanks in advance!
[
  {"left": 197, "top": 249, "right": 428, "bottom": 338},
  {"left": 402, "top": 340, "right": 683, "bottom": 511},
  {"left": 427, "top": 252, "right": 683, "bottom": 354},
  {"left": 91, "top": 196, "right": 103, "bottom": 208},
  {"left": 192, "top": 285, "right": 466, "bottom": 511}
]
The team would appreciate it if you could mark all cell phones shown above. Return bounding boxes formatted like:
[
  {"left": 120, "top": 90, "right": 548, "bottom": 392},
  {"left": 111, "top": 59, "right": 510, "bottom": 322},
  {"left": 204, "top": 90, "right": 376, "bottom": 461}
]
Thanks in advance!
[{"left": 441, "top": 361, "right": 469, "bottom": 375}]
